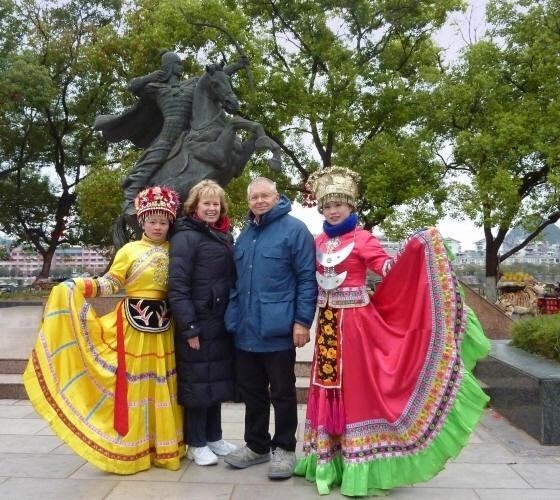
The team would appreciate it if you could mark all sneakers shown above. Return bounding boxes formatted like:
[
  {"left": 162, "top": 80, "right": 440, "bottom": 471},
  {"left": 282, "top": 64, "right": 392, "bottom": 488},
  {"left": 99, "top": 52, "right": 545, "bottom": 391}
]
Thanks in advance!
[
  {"left": 186, "top": 445, "right": 219, "bottom": 466},
  {"left": 224, "top": 443, "right": 272, "bottom": 468},
  {"left": 205, "top": 439, "right": 237, "bottom": 455},
  {"left": 268, "top": 446, "right": 296, "bottom": 478}
]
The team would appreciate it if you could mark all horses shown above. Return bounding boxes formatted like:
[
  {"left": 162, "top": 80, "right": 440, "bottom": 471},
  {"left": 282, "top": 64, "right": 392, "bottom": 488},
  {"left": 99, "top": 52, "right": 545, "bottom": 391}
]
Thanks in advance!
[{"left": 111, "top": 58, "right": 283, "bottom": 254}]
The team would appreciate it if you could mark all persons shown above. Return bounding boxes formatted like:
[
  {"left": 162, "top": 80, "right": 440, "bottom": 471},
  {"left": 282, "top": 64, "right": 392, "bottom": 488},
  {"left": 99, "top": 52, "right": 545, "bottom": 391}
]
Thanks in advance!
[
  {"left": 117, "top": 51, "right": 250, "bottom": 220},
  {"left": 293, "top": 164, "right": 492, "bottom": 499},
  {"left": 223, "top": 177, "right": 318, "bottom": 481},
  {"left": 168, "top": 177, "right": 237, "bottom": 467},
  {"left": 21, "top": 185, "right": 188, "bottom": 476}
]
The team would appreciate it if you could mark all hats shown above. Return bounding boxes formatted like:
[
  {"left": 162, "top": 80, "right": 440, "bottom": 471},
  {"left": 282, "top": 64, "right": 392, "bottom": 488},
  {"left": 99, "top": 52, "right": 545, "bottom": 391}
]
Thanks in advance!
[
  {"left": 134, "top": 186, "right": 180, "bottom": 225},
  {"left": 305, "top": 164, "right": 362, "bottom": 215}
]
